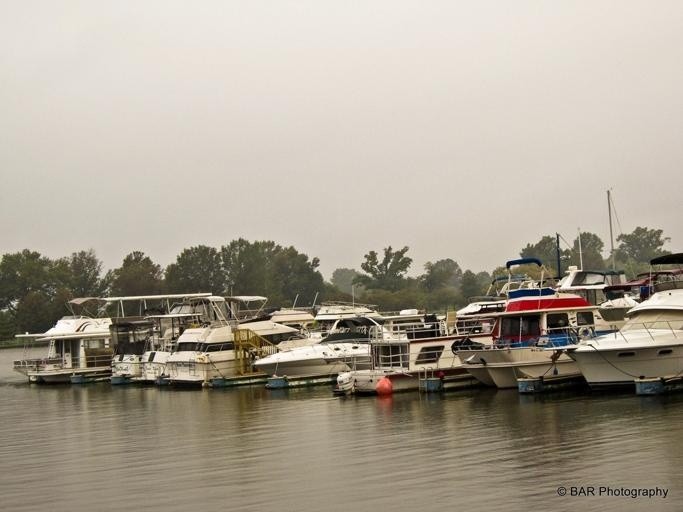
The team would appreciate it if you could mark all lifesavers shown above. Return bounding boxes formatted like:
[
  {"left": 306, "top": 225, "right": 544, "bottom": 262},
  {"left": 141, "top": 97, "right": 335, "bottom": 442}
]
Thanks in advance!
[{"left": 579, "top": 327, "right": 592, "bottom": 340}]
